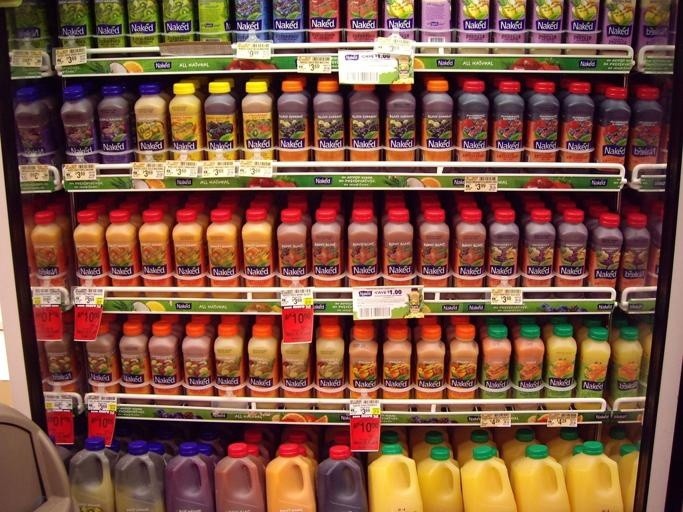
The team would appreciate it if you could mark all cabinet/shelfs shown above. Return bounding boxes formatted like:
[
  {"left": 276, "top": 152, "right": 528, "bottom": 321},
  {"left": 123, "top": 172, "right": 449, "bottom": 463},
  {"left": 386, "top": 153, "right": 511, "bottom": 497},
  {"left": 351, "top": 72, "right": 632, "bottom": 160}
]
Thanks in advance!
[
  {"left": 5, "top": 230, "right": 9, "bottom": 232},
  {"left": 63, "top": 42, "right": 637, "bottom": 425},
  {"left": 7, "top": 50, "right": 85, "bottom": 415},
  {"left": 620, "top": 46, "right": 675, "bottom": 314}
]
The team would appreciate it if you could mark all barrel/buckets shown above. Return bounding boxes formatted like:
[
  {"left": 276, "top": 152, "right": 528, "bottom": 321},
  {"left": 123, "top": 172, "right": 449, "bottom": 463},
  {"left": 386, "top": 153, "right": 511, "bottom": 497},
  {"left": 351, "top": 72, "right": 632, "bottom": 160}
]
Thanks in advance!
[
  {"left": 604, "top": 426, "right": 631, "bottom": 456},
  {"left": 113, "top": 439, "right": 167, "bottom": 512},
  {"left": 213, "top": 441, "right": 266, "bottom": 512},
  {"left": 266, "top": 444, "right": 317, "bottom": 512},
  {"left": 47, "top": 434, "right": 72, "bottom": 470},
  {"left": 417, "top": 446, "right": 463, "bottom": 512},
  {"left": 511, "top": 444, "right": 571, "bottom": 512},
  {"left": 563, "top": 441, "right": 624, "bottom": 512},
  {"left": 460, "top": 446, "right": 518, "bottom": 512},
  {"left": 156, "top": 428, "right": 178, "bottom": 452},
  {"left": 316, "top": 445, "right": 370, "bottom": 512},
  {"left": 164, "top": 441, "right": 215, "bottom": 511},
  {"left": 68, "top": 435, "right": 118, "bottom": 512},
  {"left": 366, "top": 444, "right": 423, "bottom": 512}
]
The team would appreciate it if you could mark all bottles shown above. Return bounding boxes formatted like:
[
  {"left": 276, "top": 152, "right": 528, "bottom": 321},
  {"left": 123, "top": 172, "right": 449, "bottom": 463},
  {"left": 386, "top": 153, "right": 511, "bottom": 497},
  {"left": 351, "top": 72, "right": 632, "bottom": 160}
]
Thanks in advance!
[
  {"left": 11, "top": 76, "right": 668, "bottom": 173},
  {"left": 3, "top": 0, "right": 674, "bottom": 54},
  {"left": 29, "top": 192, "right": 651, "bottom": 299},
  {"left": 43, "top": 314, "right": 216, "bottom": 395},
  {"left": 213, "top": 316, "right": 645, "bottom": 410}
]
